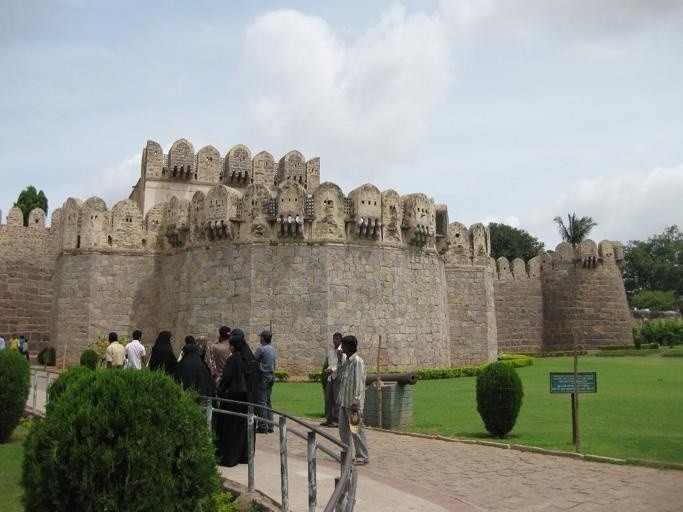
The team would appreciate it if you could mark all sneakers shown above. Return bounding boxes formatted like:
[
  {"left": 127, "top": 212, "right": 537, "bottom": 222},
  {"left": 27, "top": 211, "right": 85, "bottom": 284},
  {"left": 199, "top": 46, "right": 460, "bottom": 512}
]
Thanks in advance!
[
  {"left": 353, "top": 458, "right": 369, "bottom": 466},
  {"left": 255, "top": 427, "right": 274, "bottom": 433},
  {"left": 328, "top": 422, "right": 339, "bottom": 427},
  {"left": 320, "top": 422, "right": 328, "bottom": 426}
]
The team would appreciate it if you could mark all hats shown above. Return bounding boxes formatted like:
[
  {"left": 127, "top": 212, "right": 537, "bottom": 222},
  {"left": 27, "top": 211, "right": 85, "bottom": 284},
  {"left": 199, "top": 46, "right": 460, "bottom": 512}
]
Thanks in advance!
[
  {"left": 258, "top": 330, "right": 272, "bottom": 337},
  {"left": 348, "top": 410, "right": 361, "bottom": 433},
  {"left": 226, "top": 329, "right": 244, "bottom": 338}
]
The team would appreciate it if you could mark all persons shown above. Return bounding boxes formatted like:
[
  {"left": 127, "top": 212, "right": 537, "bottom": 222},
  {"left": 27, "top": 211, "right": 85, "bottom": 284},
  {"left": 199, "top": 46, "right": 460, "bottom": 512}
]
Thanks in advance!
[
  {"left": 124, "top": 328, "right": 148, "bottom": 372},
  {"left": 320, "top": 331, "right": 344, "bottom": 429},
  {"left": 0, "top": 333, "right": 31, "bottom": 361},
  {"left": 151, "top": 325, "right": 261, "bottom": 468},
  {"left": 104, "top": 331, "right": 127, "bottom": 372},
  {"left": 333, "top": 335, "right": 369, "bottom": 465},
  {"left": 255, "top": 328, "right": 278, "bottom": 434}
]
